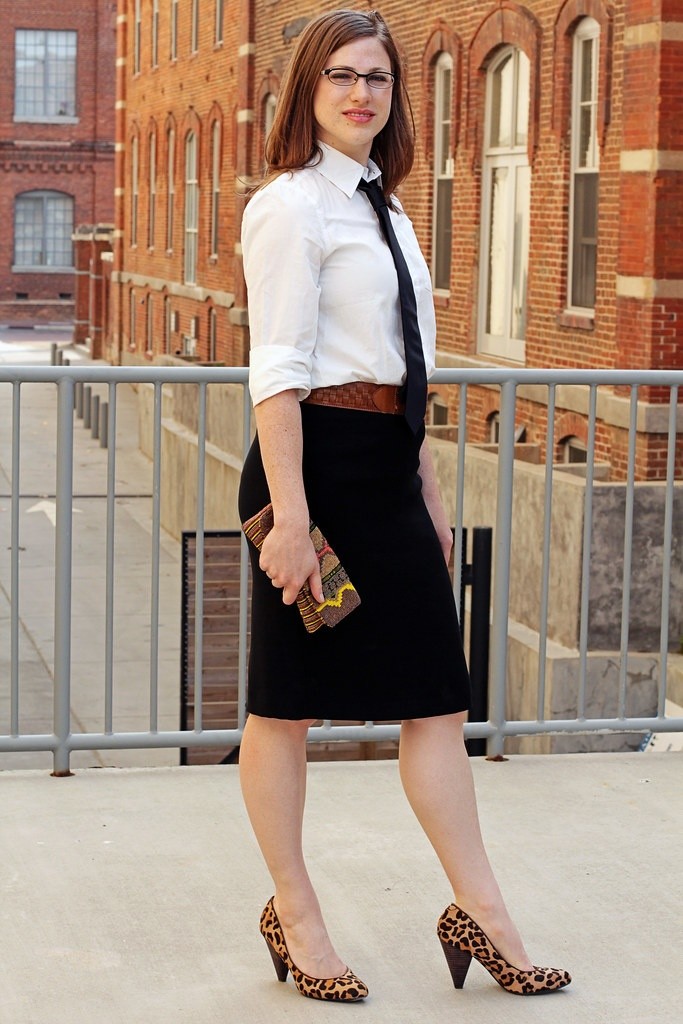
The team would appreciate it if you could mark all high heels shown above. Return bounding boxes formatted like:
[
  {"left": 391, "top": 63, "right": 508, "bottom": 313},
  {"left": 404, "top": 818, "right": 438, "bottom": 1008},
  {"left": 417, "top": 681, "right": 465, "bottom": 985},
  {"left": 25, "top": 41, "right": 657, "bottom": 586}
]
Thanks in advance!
[
  {"left": 258, "top": 895, "right": 369, "bottom": 1002},
  {"left": 434, "top": 903, "right": 573, "bottom": 995}
]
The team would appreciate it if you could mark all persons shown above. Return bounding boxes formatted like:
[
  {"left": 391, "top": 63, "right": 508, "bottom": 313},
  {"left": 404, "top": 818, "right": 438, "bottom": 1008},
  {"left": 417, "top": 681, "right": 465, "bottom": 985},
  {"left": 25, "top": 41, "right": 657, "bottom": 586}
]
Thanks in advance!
[{"left": 238, "top": 11, "right": 570, "bottom": 1002}]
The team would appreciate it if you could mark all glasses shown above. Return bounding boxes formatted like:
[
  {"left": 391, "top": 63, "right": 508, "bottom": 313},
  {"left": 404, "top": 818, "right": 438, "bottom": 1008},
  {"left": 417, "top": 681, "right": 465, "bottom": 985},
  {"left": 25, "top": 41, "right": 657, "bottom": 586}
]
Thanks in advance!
[{"left": 320, "top": 68, "right": 395, "bottom": 89}]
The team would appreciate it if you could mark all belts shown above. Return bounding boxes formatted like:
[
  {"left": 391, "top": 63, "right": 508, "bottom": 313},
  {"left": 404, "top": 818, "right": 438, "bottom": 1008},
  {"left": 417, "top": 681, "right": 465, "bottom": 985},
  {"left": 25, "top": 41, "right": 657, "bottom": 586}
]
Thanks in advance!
[{"left": 304, "top": 380, "right": 402, "bottom": 415}]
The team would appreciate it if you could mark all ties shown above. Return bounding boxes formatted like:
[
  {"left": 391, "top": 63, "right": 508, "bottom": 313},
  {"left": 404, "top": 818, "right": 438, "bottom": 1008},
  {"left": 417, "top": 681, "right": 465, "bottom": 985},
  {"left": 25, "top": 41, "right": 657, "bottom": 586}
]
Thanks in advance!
[{"left": 356, "top": 178, "right": 427, "bottom": 463}]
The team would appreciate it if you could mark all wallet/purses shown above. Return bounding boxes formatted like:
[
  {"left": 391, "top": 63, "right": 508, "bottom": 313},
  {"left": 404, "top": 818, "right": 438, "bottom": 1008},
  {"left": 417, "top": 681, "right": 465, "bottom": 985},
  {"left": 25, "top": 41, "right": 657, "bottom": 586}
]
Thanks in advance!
[{"left": 241, "top": 501, "right": 362, "bottom": 633}]
